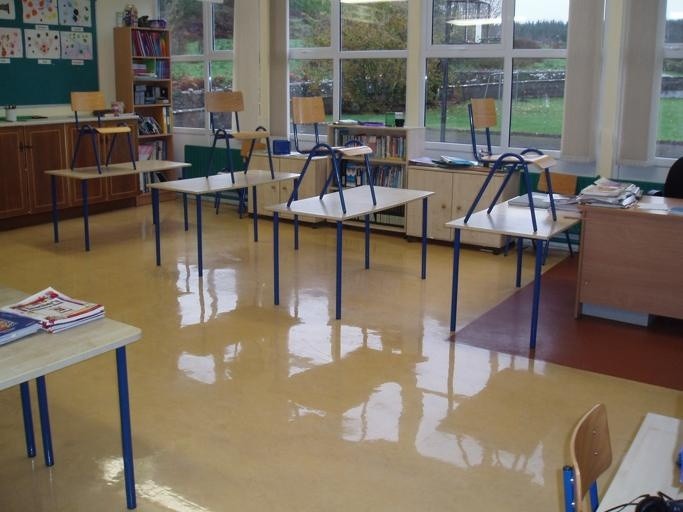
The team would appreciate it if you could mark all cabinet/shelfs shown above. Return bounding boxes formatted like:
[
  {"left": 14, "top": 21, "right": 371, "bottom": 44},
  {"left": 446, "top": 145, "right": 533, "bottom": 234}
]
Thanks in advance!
[
  {"left": 113, "top": 27, "right": 177, "bottom": 205},
  {"left": 0, "top": 119, "right": 141, "bottom": 232},
  {"left": 246, "top": 151, "right": 327, "bottom": 225},
  {"left": 327, "top": 123, "right": 426, "bottom": 238},
  {"left": 406, "top": 165, "right": 520, "bottom": 255}
]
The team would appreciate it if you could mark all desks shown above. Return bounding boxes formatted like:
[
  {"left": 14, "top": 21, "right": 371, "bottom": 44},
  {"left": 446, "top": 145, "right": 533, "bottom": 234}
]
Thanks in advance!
[
  {"left": 574, "top": 196, "right": 683, "bottom": 321},
  {"left": 596, "top": 412, "right": 683, "bottom": 512},
  {"left": 146, "top": 170, "right": 303, "bottom": 277},
  {"left": 264, "top": 185, "right": 436, "bottom": 319},
  {"left": 443, "top": 191, "right": 583, "bottom": 348},
  {"left": 44, "top": 160, "right": 192, "bottom": 252},
  {"left": 0, "top": 286, "right": 144, "bottom": 509}
]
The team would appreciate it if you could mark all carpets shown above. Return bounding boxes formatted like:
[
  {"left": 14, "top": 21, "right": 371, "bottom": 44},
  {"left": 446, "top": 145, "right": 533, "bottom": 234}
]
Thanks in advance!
[{"left": 444, "top": 251, "right": 683, "bottom": 392}]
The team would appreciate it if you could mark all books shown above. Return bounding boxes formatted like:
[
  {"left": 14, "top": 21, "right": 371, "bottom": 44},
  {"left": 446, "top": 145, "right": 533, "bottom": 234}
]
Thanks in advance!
[
  {"left": 330, "top": 119, "right": 407, "bottom": 229},
  {"left": 123, "top": 4, "right": 173, "bottom": 194},
  {"left": 0, "top": 285, "right": 106, "bottom": 347},
  {"left": 546, "top": 176, "right": 644, "bottom": 218},
  {"left": 406, "top": 155, "right": 475, "bottom": 169},
  {"left": 508, "top": 191, "right": 552, "bottom": 210}
]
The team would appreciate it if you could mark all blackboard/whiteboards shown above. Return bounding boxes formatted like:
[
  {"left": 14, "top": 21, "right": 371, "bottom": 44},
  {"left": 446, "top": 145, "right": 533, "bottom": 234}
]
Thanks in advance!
[{"left": 0, "top": 0, "right": 101, "bottom": 109}]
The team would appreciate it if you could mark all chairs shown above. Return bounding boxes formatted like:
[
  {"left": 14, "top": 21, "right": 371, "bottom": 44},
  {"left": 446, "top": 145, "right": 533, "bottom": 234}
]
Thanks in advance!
[
  {"left": 464, "top": 97, "right": 557, "bottom": 231},
  {"left": 664, "top": 156, "right": 683, "bottom": 199},
  {"left": 503, "top": 172, "right": 578, "bottom": 265},
  {"left": 204, "top": 91, "right": 274, "bottom": 184},
  {"left": 70, "top": 90, "right": 137, "bottom": 173},
  {"left": 287, "top": 97, "right": 377, "bottom": 214},
  {"left": 563, "top": 402, "right": 613, "bottom": 512},
  {"left": 214, "top": 140, "right": 266, "bottom": 219}
]
